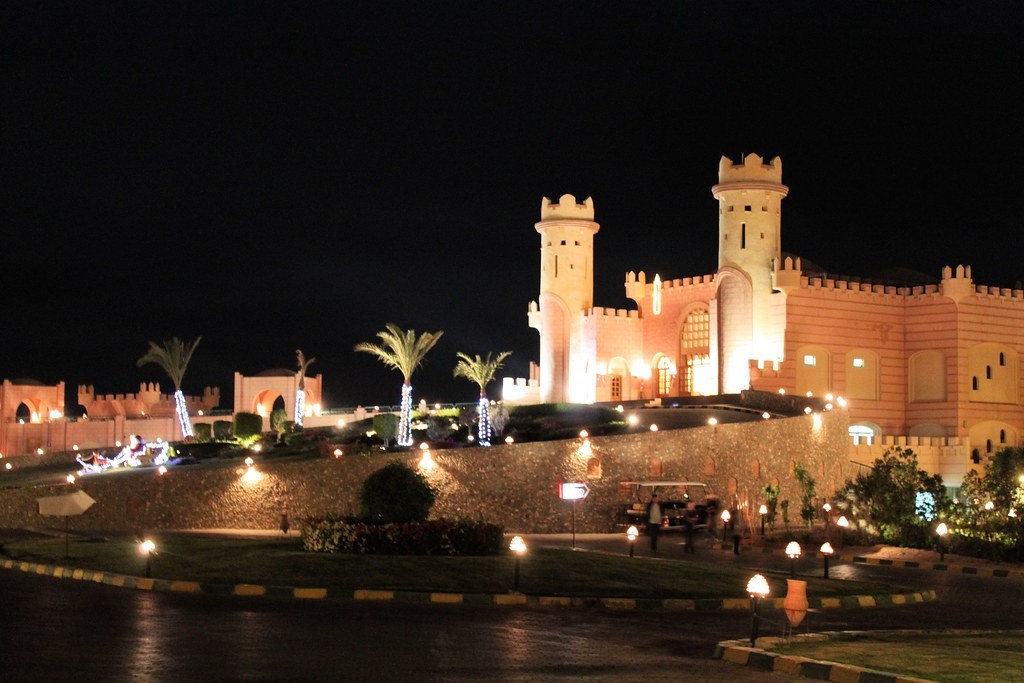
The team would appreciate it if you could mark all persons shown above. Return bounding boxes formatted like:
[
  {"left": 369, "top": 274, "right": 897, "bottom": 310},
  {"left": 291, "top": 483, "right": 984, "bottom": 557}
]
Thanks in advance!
[
  {"left": 645, "top": 494, "right": 664, "bottom": 555},
  {"left": 729, "top": 509, "right": 743, "bottom": 556},
  {"left": 76, "top": 433, "right": 145, "bottom": 473},
  {"left": 682, "top": 500, "right": 698, "bottom": 553},
  {"left": 702, "top": 500, "right": 720, "bottom": 548}
]
